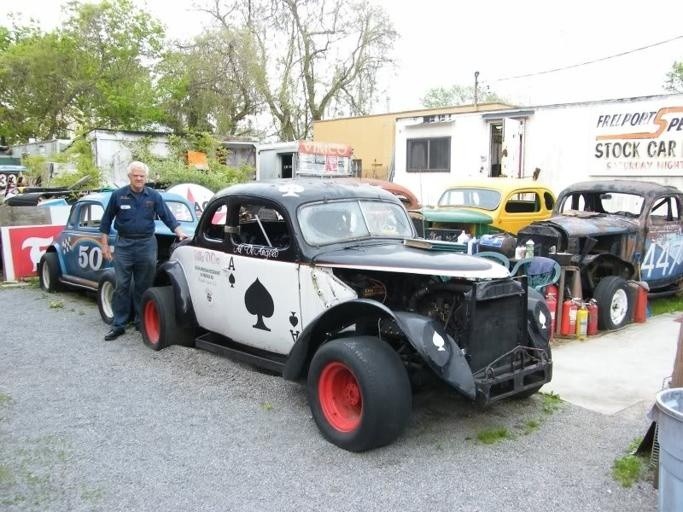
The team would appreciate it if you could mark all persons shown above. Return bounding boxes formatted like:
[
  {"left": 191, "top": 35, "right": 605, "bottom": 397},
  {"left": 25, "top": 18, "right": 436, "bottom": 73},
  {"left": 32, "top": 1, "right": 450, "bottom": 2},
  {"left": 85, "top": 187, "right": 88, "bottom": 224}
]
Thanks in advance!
[{"left": 99, "top": 161, "right": 189, "bottom": 341}]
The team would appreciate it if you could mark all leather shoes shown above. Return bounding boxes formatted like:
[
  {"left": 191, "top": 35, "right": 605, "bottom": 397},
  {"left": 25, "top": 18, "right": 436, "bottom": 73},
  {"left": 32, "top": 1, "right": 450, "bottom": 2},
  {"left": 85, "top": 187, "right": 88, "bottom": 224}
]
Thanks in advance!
[{"left": 104, "top": 327, "right": 125, "bottom": 340}]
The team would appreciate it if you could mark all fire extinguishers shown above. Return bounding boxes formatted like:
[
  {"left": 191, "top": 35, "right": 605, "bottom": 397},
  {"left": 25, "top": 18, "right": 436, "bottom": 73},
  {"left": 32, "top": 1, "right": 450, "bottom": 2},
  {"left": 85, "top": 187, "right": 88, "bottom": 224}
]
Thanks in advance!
[
  {"left": 545, "top": 293, "right": 556, "bottom": 339},
  {"left": 567, "top": 298, "right": 581, "bottom": 336},
  {"left": 588, "top": 298, "right": 598, "bottom": 335},
  {"left": 576, "top": 303, "right": 590, "bottom": 339}
]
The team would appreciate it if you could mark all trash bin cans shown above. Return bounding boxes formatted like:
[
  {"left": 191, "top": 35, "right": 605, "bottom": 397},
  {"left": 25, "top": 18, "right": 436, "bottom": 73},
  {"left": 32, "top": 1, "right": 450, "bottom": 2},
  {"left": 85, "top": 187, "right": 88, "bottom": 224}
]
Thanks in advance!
[{"left": 654, "top": 387, "right": 683, "bottom": 511}]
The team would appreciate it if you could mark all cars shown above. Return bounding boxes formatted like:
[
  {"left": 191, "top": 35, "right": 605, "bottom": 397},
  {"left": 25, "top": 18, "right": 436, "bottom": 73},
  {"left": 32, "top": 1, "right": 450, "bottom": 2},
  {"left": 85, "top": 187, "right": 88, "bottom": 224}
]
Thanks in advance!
[
  {"left": 139, "top": 177, "right": 554, "bottom": 452},
  {"left": 475, "top": 179, "right": 683, "bottom": 333},
  {"left": 36, "top": 191, "right": 199, "bottom": 325},
  {"left": 408, "top": 181, "right": 558, "bottom": 243}
]
